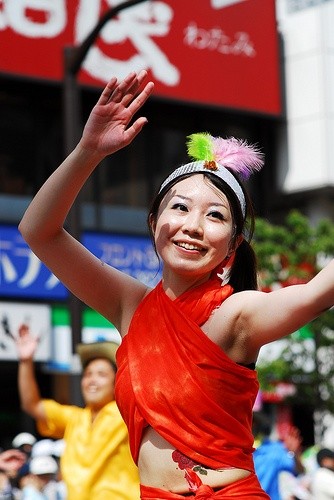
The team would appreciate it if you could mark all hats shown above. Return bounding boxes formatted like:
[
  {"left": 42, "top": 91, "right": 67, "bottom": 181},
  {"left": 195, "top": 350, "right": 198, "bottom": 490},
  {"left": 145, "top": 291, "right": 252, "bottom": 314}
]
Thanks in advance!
[{"left": 76, "top": 340, "right": 121, "bottom": 369}]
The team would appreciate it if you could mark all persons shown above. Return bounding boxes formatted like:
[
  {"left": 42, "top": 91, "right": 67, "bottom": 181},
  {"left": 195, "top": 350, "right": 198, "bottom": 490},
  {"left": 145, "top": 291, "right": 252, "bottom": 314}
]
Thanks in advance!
[
  {"left": 19, "top": 66, "right": 334, "bottom": 500},
  {"left": 251, "top": 413, "right": 304, "bottom": 500},
  {"left": 298, "top": 448, "right": 334, "bottom": 500},
  {"left": 0, "top": 432, "right": 68, "bottom": 500},
  {"left": 13, "top": 325, "right": 140, "bottom": 500}
]
